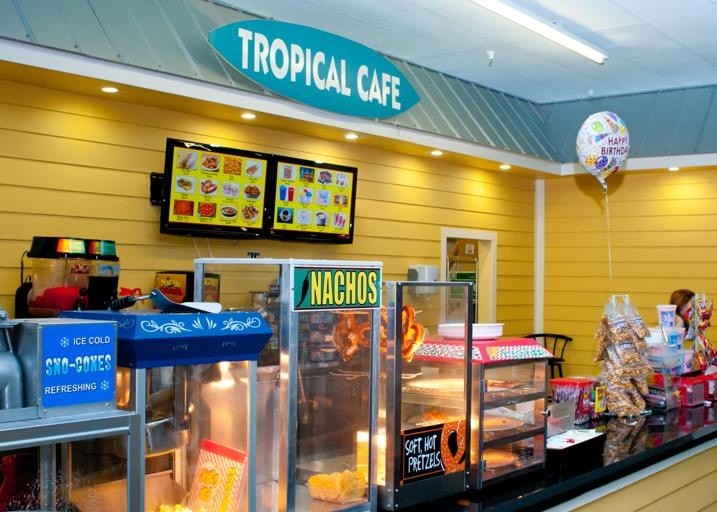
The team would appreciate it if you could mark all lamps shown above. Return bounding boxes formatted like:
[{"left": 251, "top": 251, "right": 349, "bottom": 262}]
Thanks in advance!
[{"left": 474, "top": 1, "right": 608, "bottom": 66}]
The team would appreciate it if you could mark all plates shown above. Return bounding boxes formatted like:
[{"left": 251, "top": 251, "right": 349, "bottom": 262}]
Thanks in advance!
[
  {"left": 408, "top": 377, "right": 510, "bottom": 392},
  {"left": 438, "top": 323, "right": 504, "bottom": 340},
  {"left": 483, "top": 449, "right": 519, "bottom": 469},
  {"left": 484, "top": 414, "right": 525, "bottom": 432}
]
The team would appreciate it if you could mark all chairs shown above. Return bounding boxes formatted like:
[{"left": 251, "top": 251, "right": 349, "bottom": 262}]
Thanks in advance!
[{"left": 525, "top": 333, "right": 573, "bottom": 403}]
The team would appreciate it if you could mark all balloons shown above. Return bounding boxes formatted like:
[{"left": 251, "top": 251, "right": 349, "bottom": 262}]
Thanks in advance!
[{"left": 575, "top": 111, "right": 631, "bottom": 192}]
